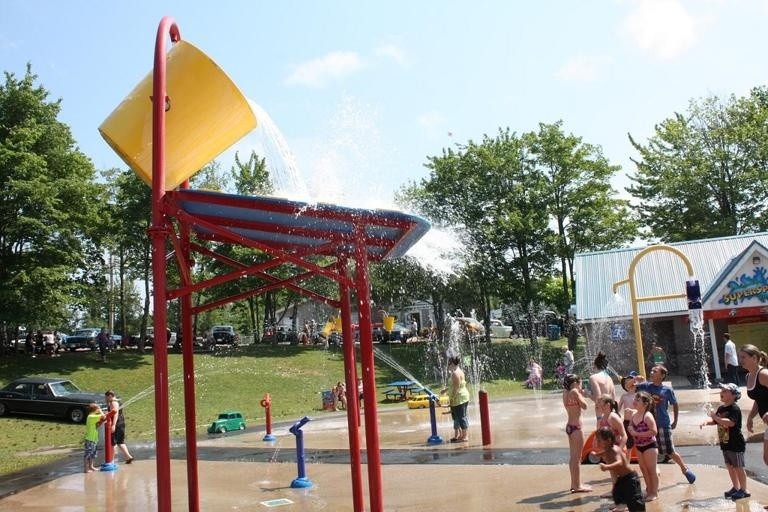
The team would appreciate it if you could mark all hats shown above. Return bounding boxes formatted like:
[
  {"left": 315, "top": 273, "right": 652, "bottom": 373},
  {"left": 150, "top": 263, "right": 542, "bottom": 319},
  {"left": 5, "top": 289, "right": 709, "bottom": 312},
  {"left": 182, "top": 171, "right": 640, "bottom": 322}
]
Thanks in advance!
[{"left": 719, "top": 381, "right": 742, "bottom": 400}]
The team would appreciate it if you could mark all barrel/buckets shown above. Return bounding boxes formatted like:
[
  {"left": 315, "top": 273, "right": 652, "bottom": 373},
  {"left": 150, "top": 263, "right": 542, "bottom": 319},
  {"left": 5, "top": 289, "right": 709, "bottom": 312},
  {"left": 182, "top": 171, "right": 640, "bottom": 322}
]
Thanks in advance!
[{"left": 97, "top": 38, "right": 258, "bottom": 192}]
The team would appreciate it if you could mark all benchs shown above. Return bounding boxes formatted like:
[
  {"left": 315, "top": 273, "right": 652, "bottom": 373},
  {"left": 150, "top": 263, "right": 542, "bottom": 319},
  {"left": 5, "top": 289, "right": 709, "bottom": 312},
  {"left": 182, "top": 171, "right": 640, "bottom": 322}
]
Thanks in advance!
[
  {"left": 382, "top": 387, "right": 424, "bottom": 395},
  {"left": 321, "top": 390, "right": 343, "bottom": 409}
]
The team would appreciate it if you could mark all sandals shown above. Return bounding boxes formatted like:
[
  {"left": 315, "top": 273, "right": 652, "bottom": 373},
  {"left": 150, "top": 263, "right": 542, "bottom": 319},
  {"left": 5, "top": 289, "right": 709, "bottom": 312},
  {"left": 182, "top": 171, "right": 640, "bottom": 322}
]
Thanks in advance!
[
  {"left": 682, "top": 465, "right": 696, "bottom": 485},
  {"left": 724, "top": 488, "right": 752, "bottom": 501}
]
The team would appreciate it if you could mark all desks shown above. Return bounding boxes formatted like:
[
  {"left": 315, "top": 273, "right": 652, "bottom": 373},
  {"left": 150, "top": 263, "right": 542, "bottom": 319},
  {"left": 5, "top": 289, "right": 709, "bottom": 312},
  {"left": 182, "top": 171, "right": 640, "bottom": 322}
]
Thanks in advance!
[{"left": 387, "top": 380, "right": 420, "bottom": 403}]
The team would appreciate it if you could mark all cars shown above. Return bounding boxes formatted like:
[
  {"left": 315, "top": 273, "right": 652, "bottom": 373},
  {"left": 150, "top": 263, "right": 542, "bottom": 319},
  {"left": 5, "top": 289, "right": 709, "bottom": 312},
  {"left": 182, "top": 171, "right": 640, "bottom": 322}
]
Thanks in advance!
[
  {"left": 0, "top": 376, "right": 122, "bottom": 427},
  {"left": 431, "top": 308, "right": 578, "bottom": 342}
]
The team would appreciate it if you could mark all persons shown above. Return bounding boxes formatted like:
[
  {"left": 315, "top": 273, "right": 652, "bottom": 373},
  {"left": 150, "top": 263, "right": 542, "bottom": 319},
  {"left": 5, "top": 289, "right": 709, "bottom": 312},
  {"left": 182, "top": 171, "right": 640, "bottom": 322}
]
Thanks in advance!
[
  {"left": 105, "top": 390, "right": 134, "bottom": 464},
  {"left": 357, "top": 377, "right": 364, "bottom": 400},
  {"left": 525, "top": 313, "right": 768, "bottom": 512},
  {"left": 1, "top": 313, "right": 493, "bottom": 379},
  {"left": 333, "top": 382, "right": 347, "bottom": 411},
  {"left": 440, "top": 355, "right": 470, "bottom": 443},
  {"left": 83, "top": 403, "right": 105, "bottom": 473},
  {"left": 439, "top": 404, "right": 462, "bottom": 442}
]
